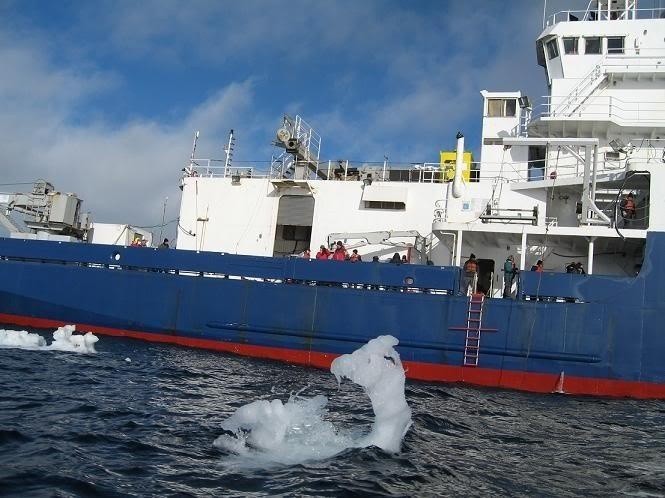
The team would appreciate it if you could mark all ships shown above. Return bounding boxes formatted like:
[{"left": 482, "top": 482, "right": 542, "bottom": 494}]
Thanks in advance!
[{"left": 0, "top": 0, "right": 664, "bottom": 401}]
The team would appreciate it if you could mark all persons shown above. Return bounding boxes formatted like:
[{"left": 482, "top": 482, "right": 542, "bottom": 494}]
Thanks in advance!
[
  {"left": 298, "top": 241, "right": 407, "bottom": 303},
  {"left": 504, "top": 255, "right": 516, "bottom": 297},
  {"left": 565, "top": 262, "right": 577, "bottom": 303},
  {"left": 531, "top": 260, "right": 543, "bottom": 302},
  {"left": 634, "top": 264, "right": 641, "bottom": 277},
  {"left": 129, "top": 238, "right": 142, "bottom": 270},
  {"left": 573, "top": 262, "right": 585, "bottom": 275},
  {"left": 159, "top": 239, "right": 170, "bottom": 273},
  {"left": 620, "top": 193, "right": 636, "bottom": 229},
  {"left": 463, "top": 254, "right": 479, "bottom": 296}
]
